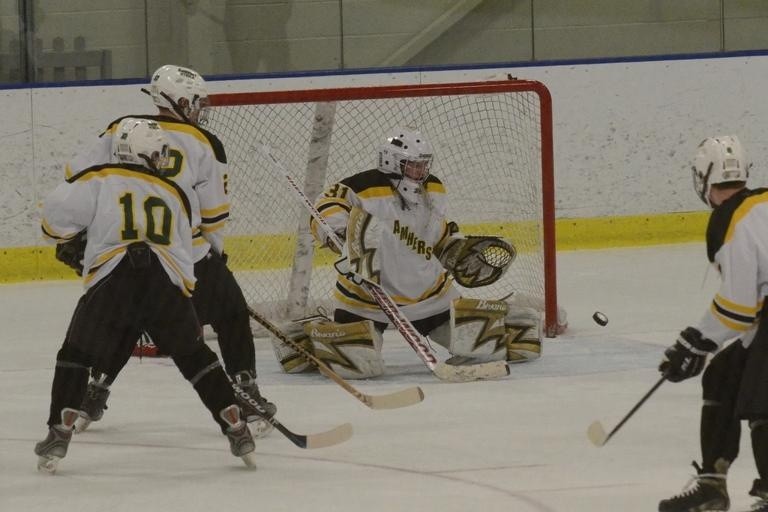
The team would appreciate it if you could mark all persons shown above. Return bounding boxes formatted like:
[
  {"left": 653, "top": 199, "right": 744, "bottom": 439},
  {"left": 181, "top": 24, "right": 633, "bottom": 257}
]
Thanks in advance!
[
  {"left": 271, "top": 124, "right": 543, "bottom": 380},
  {"left": 35, "top": 116, "right": 256, "bottom": 459},
  {"left": 63, "top": 62, "right": 277, "bottom": 423},
  {"left": 654, "top": 133, "right": 768, "bottom": 511}
]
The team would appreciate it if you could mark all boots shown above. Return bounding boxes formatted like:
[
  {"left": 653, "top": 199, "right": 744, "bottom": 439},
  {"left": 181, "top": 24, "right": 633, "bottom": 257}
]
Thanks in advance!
[
  {"left": 220, "top": 406, "right": 255, "bottom": 455},
  {"left": 658, "top": 458, "right": 732, "bottom": 511},
  {"left": 34, "top": 408, "right": 81, "bottom": 458},
  {"left": 231, "top": 371, "right": 277, "bottom": 421},
  {"left": 79, "top": 373, "right": 112, "bottom": 420}
]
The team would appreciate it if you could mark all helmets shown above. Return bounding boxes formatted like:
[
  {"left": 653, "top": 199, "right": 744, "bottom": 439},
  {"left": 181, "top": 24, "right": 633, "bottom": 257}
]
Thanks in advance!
[
  {"left": 693, "top": 134, "right": 748, "bottom": 209},
  {"left": 152, "top": 65, "right": 212, "bottom": 128},
  {"left": 377, "top": 127, "right": 433, "bottom": 204},
  {"left": 111, "top": 118, "right": 169, "bottom": 176}
]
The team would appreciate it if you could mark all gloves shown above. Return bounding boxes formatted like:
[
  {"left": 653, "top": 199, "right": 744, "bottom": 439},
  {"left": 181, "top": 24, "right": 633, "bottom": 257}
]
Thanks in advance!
[
  {"left": 659, "top": 327, "right": 719, "bottom": 382},
  {"left": 56, "top": 228, "right": 88, "bottom": 276}
]
